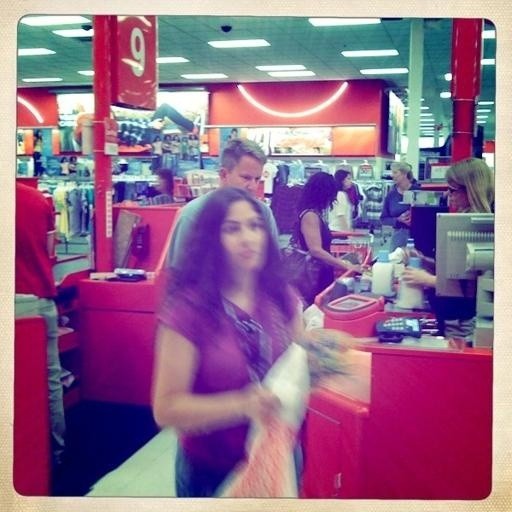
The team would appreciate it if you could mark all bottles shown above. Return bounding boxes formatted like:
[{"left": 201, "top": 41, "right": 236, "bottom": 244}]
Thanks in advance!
[
  {"left": 398, "top": 256, "right": 425, "bottom": 308},
  {"left": 371, "top": 250, "right": 395, "bottom": 296},
  {"left": 405, "top": 238, "right": 415, "bottom": 253},
  {"left": 242, "top": 332, "right": 313, "bottom": 460}
]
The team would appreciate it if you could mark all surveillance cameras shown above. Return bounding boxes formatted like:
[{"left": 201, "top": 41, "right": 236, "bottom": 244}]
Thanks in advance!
[
  {"left": 220, "top": 27, "right": 232, "bottom": 32},
  {"left": 82, "top": 25, "right": 91, "bottom": 31}
]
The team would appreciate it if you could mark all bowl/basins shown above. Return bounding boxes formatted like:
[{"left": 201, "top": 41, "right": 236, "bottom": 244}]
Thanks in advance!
[{"left": 114, "top": 267, "right": 146, "bottom": 281}]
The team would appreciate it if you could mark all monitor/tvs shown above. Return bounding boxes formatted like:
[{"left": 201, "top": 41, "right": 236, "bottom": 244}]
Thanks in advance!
[{"left": 435, "top": 213, "right": 495, "bottom": 299}]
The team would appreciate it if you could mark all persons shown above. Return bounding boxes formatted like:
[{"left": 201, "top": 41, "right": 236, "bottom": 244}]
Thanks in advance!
[
  {"left": 60, "top": 157, "right": 71, "bottom": 175},
  {"left": 148, "top": 184, "right": 359, "bottom": 497},
  {"left": 325, "top": 169, "right": 355, "bottom": 234},
  {"left": 116, "top": 104, "right": 201, "bottom": 147},
  {"left": 379, "top": 161, "right": 423, "bottom": 253},
  {"left": 400, "top": 157, "right": 494, "bottom": 347},
  {"left": 69, "top": 156, "right": 78, "bottom": 176},
  {"left": 15, "top": 178, "right": 70, "bottom": 470},
  {"left": 158, "top": 139, "right": 284, "bottom": 283},
  {"left": 146, "top": 168, "right": 175, "bottom": 205},
  {"left": 34, "top": 129, "right": 45, "bottom": 179},
  {"left": 287, "top": 172, "right": 366, "bottom": 306}
]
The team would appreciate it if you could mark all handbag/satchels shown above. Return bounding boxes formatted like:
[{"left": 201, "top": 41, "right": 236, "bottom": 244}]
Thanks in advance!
[{"left": 280, "top": 245, "right": 322, "bottom": 300}]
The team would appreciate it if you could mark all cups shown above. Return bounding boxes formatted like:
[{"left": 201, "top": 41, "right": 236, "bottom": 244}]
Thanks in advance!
[
  {"left": 416, "top": 190, "right": 428, "bottom": 205},
  {"left": 402, "top": 190, "right": 416, "bottom": 204},
  {"left": 288, "top": 247, "right": 309, "bottom": 272}
]
